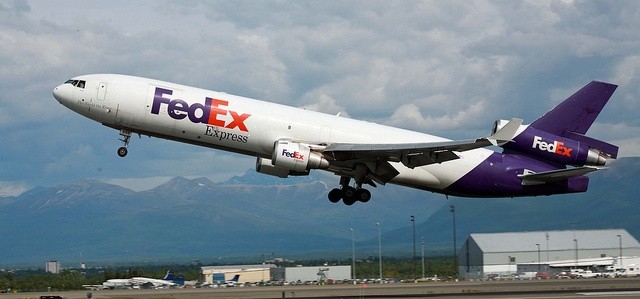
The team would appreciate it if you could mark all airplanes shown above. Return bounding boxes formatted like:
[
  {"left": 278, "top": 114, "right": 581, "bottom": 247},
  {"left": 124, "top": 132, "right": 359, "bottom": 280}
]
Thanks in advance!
[
  {"left": 51, "top": 71, "right": 623, "bottom": 208},
  {"left": 102, "top": 267, "right": 174, "bottom": 291},
  {"left": 197, "top": 273, "right": 240, "bottom": 288},
  {"left": 129, "top": 271, "right": 184, "bottom": 291}
]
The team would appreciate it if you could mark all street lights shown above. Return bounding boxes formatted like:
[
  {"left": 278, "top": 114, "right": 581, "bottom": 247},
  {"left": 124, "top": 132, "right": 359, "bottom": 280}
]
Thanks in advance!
[
  {"left": 536, "top": 243, "right": 540, "bottom": 272},
  {"left": 450, "top": 208, "right": 457, "bottom": 273},
  {"left": 410, "top": 216, "right": 416, "bottom": 277},
  {"left": 573, "top": 239, "right": 578, "bottom": 269},
  {"left": 349, "top": 228, "right": 355, "bottom": 280},
  {"left": 617, "top": 234, "right": 622, "bottom": 267},
  {"left": 376, "top": 222, "right": 382, "bottom": 281}
]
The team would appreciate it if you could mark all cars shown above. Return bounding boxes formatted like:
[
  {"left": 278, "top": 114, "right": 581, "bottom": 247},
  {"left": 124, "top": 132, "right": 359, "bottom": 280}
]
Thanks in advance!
[
  {"left": 482, "top": 264, "right": 640, "bottom": 282},
  {"left": 243, "top": 278, "right": 395, "bottom": 286}
]
